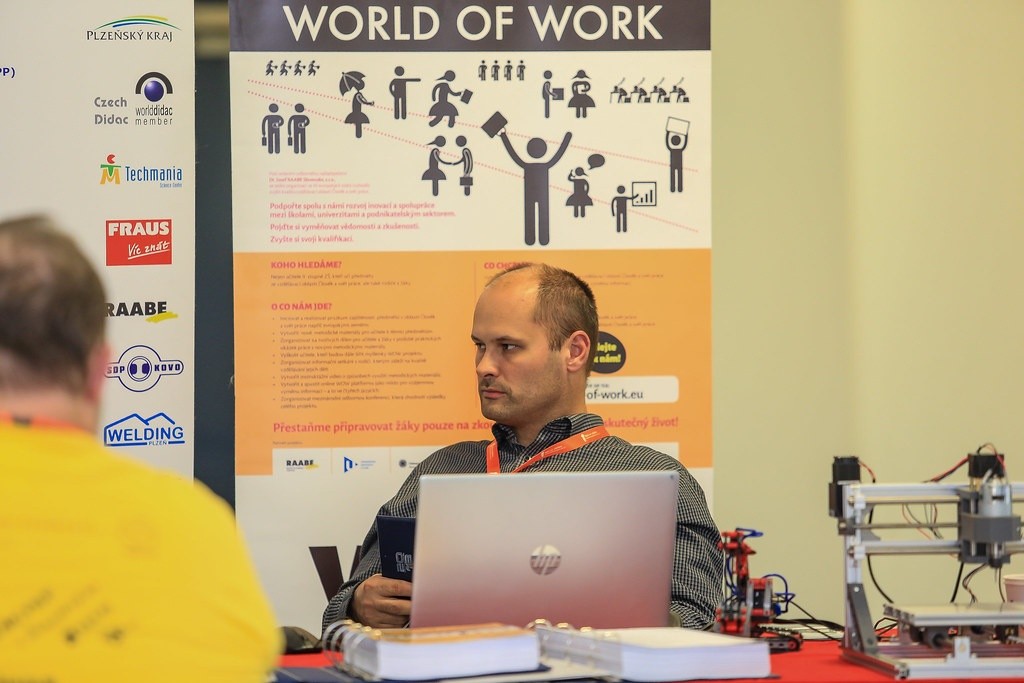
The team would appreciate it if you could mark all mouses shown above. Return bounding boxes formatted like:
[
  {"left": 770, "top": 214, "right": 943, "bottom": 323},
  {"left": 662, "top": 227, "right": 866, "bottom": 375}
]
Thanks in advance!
[{"left": 281, "top": 626, "right": 322, "bottom": 655}]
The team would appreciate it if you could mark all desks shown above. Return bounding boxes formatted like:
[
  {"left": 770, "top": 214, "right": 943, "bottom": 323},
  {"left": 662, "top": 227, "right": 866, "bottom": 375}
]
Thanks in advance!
[{"left": 279, "top": 629, "right": 1024, "bottom": 683}]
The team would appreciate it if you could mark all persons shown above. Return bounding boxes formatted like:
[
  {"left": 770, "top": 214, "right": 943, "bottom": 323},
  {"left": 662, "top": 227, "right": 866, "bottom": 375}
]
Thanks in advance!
[
  {"left": 0, "top": 213, "right": 286, "bottom": 683},
  {"left": 322, "top": 261, "right": 722, "bottom": 631}
]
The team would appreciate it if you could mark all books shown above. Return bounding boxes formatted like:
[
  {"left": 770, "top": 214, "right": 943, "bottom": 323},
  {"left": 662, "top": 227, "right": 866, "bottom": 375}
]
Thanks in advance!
[
  {"left": 526, "top": 619, "right": 773, "bottom": 683},
  {"left": 320, "top": 620, "right": 541, "bottom": 683}
]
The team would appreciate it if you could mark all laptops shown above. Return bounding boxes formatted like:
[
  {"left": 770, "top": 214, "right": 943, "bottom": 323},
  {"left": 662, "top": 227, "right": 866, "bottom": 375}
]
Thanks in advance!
[{"left": 410, "top": 471, "right": 680, "bottom": 630}]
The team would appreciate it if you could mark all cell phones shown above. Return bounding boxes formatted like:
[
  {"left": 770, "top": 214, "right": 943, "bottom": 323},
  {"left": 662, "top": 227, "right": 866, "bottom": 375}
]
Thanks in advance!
[{"left": 752, "top": 632, "right": 799, "bottom": 651}]
